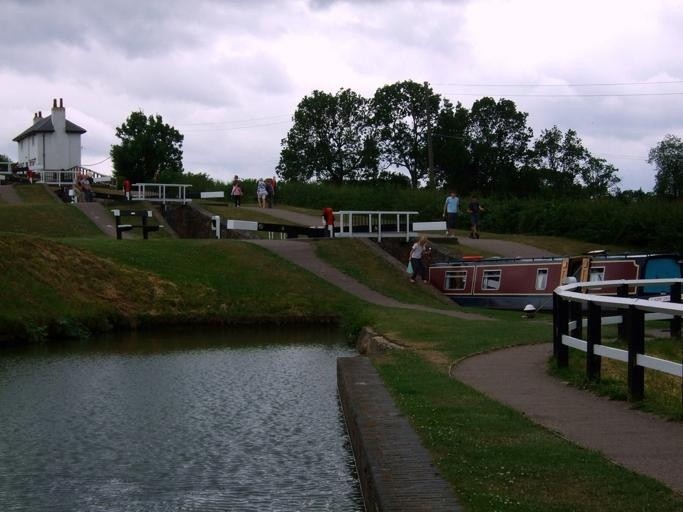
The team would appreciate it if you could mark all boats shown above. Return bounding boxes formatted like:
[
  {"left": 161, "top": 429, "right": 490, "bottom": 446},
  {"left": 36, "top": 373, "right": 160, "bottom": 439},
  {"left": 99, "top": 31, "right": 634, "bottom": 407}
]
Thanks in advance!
[{"left": 418, "top": 247, "right": 683, "bottom": 333}]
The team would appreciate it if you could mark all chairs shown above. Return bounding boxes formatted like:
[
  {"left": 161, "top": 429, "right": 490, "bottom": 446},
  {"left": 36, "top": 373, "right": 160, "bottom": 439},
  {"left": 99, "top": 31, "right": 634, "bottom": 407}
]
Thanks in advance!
[{"left": 111, "top": 207, "right": 167, "bottom": 240}]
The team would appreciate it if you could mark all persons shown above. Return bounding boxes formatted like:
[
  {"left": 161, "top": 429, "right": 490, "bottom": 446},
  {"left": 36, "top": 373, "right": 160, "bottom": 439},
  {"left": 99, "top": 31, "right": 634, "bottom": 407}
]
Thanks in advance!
[
  {"left": 256, "top": 176, "right": 276, "bottom": 209},
  {"left": 72, "top": 173, "right": 97, "bottom": 203},
  {"left": 466, "top": 196, "right": 485, "bottom": 239},
  {"left": 26, "top": 170, "right": 33, "bottom": 184},
  {"left": 443, "top": 192, "right": 465, "bottom": 236},
  {"left": 123, "top": 180, "right": 131, "bottom": 201},
  {"left": 321, "top": 207, "right": 335, "bottom": 230},
  {"left": 231, "top": 175, "right": 243, "bottom": 208},
  {"left": 409, "top": 236, "right": 429, "bottom": 284}
]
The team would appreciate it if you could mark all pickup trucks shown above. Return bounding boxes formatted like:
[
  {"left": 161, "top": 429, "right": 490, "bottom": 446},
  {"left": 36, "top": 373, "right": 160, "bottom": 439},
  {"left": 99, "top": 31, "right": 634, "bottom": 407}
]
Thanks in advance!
[{"left": 0, "top": 161, "right": 40, "bottom": 182}]
